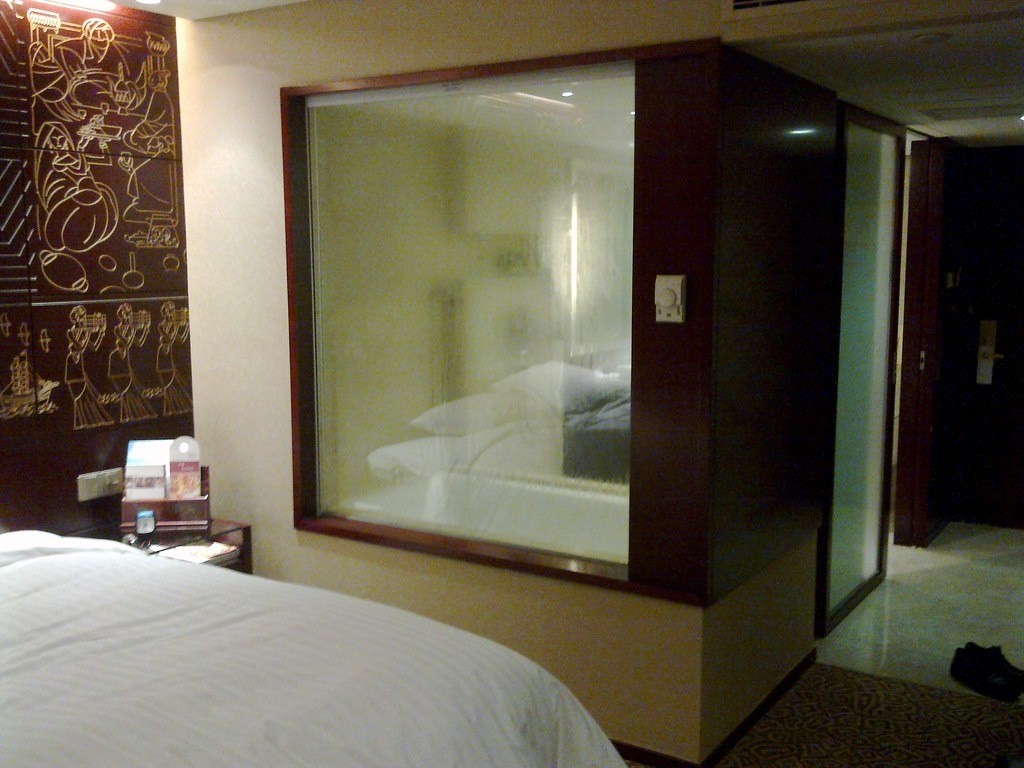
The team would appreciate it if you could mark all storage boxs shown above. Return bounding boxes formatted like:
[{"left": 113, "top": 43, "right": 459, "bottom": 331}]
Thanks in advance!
[{"left": 120, "top": 496, "right": 211, "bottom": 538}]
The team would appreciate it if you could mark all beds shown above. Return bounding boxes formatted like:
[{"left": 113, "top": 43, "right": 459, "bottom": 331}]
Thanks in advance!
[
  {"left": 1, "top": 531, "right": 628, "bottom": 768},
  {"left": 356, "top": 354, "right": 630, "bottom": 572}
]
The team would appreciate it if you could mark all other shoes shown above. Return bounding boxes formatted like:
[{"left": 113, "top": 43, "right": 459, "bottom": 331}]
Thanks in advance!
[{"left": 949, "top": 641, "right": 1024, "bottom": 703}]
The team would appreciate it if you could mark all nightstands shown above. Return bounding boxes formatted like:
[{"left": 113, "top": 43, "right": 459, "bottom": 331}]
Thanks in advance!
[{"left": 106, "top": 516, "right": 252, "bottom": 575}]
